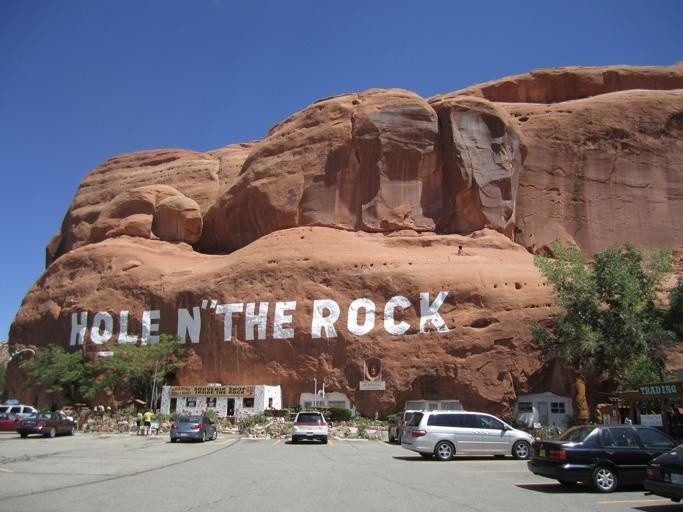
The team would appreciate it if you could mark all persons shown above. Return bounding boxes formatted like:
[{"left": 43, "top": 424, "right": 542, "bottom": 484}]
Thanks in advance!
[
  {"left": 143, "top": 409, "right": 154, "bottom": 435},
  {"left": 136, "top": 411, "right": 142, "bottom": 435}
]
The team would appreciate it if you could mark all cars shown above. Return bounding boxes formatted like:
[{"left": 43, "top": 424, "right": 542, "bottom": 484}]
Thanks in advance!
[
  {"left": 0, "top": 411, "right": 23, "bottom": 430},
  {"left": 642, "top": 444, "right": 683, "bottom": 502},
  {"left": 17, "top": 412, "right": 75, "bottom": 439},
  {"left": 527, "top": 422, "right": 679, "bottom": 492},
  {"left": 171, "top": 416, "right": 217, "bottom": 442}
]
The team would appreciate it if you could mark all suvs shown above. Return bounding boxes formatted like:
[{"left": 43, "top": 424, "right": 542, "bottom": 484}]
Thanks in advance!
[
  {"left": 1, "top": 404, "right": 37, "bottom": 418},
  {"left": 291, "top": 412, "right": 328, "bottom": 444},
  {"left": 388, "top": 410, "right": 423, "bottom": 444}
]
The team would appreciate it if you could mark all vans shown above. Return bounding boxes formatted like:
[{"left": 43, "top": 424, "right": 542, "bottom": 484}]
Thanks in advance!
[{"left": 400, "top": 409, "right": 537, "bottom": 460}]
